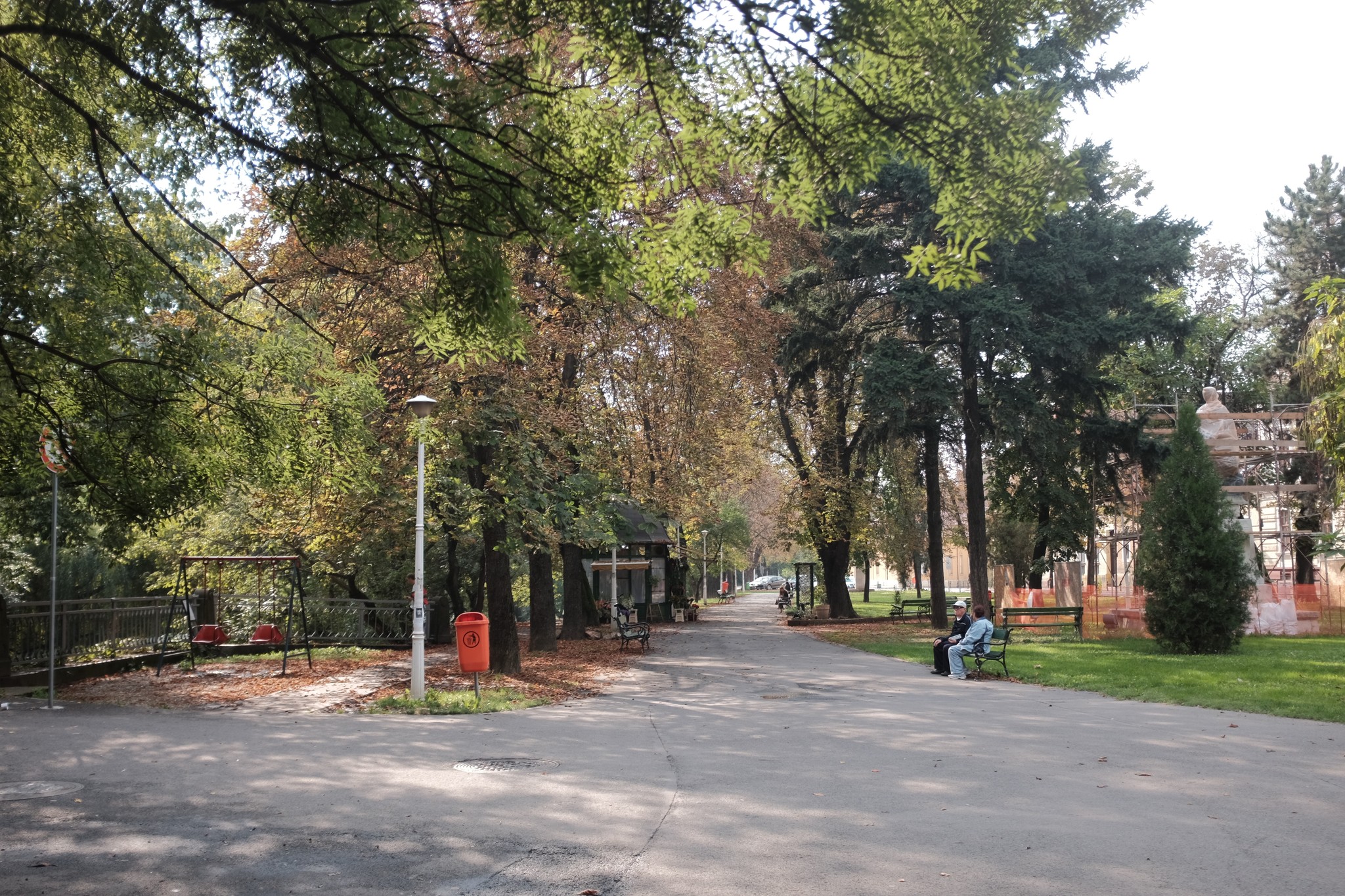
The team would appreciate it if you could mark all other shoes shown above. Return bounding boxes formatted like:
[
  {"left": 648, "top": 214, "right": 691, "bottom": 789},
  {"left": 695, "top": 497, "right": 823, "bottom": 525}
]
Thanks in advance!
[
  {"left": 941, "top": 670, "right": 951, "bottom": 676},
  {"left": 931, "top": 669, "right": 943, "bottom": 674}
]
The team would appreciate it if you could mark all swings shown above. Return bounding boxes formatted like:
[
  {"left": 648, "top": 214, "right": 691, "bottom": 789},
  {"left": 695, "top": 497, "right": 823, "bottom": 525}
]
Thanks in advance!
[
  {"left": 191, "top": 565, "right": 231, "bottom": 646},
  {"left": 247, "top": 565, "right": 286, "bottom": 644}
]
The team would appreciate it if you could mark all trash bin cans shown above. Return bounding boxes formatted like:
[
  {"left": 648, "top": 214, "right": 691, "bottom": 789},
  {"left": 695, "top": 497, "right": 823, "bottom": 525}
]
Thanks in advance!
[
  {"left": 747, "top": 582, "right": 750, "bottom": 590},
  {"left": 722, "top": 581, "right": 729, "bottom": 593},
  {"left": 453, "top": 611, "right": 494, "bottom": 671}
]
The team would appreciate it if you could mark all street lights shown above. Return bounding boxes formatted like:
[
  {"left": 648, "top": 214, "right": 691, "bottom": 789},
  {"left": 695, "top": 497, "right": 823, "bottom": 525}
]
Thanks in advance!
[
  {"left": 718, "top": 538, "right": 723, "bottom": 590},
  {"left": 700, "top": 529, "right": 708, "bottom": 606},
  {"left": 404, "top": 394, "right": 438, "bottom": 706},
  {"left": 674, "top": 514, "right": 682, "bottom": 561},
  {"left": 732, "top": 547, "right": 737, "bottom": 596}
]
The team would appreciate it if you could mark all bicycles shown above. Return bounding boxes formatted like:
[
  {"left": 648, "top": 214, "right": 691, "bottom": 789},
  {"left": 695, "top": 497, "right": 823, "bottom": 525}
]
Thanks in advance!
[{"left": 602, "top": 592, "right": 626, "bottom": 634}]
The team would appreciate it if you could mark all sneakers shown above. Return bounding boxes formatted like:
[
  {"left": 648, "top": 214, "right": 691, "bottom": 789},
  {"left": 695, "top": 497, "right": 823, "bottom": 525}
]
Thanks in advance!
[
  {"left": 964, "top": 668, "right": 972, "bottom": 675},
  {"left": 948, "top": 672, "right": 967, "bottom": 679}
]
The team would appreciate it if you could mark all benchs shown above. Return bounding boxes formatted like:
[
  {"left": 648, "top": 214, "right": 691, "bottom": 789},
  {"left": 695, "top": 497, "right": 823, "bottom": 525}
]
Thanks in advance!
[
  {"left": 716, "top": 590, "right": 731, "bottom": 604},
  {"left": 946, "top": 596, "right": 995, "bottom": 624},
  {"left": 777, "top": 588, "right": 794, "bottom": 614},
  {"left": 1000, "top": 607, "right": 1084, "bottom": 644},
  {"left": 613, "top": 613, "right": 650, "bottom": 653},
  {"left": 725, "top": 590, "right": 736, "bottom": 603},
  {"left": 889, "top": 599, "right": 931, "bottom": 625},
  {"left": 964, "top": 627, "right": 1013, "bottom": 681}
]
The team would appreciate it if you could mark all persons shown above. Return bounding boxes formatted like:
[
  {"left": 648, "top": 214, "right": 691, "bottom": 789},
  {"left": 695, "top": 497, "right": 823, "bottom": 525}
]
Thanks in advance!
[
  {"left": 775, "top": 582, "right": 794, "bottom": 610},
  {"left": 930, "top": 601, "right": 973, "bottom": 676},
  {"left": 948, "top": 605, "right": 994, "bottom": 679}
]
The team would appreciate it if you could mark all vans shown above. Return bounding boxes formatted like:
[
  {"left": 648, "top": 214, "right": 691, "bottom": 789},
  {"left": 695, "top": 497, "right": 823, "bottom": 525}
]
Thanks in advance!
[{"left": 782, "top": 573, "right": 818, "bottom": 591}]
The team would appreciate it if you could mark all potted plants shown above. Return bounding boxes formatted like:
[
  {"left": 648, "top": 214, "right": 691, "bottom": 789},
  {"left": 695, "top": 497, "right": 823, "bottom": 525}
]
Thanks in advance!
[
  {"left": 814, "top": 585, "right": 830, "bottom": 619},
  {"left": 620, "top": 594, "right": 638, "bottom": 623},
  {"left": 668, "top": 594, "right": 686, "bottom": 622}
]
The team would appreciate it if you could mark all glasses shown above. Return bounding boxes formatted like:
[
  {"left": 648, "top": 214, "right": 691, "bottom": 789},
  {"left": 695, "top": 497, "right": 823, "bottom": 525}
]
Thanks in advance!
[{"left": 953, "top": 606, "right": 964, "bottom": 609}]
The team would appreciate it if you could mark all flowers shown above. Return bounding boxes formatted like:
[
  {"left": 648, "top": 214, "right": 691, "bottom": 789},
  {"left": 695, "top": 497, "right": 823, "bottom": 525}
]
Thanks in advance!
[{"left": 692, "top": 604, "right": 699, "bottom": 609}]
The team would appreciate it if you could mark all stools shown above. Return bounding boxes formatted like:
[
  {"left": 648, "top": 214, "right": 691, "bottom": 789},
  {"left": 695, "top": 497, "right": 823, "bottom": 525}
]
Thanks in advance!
[{"left": 686, "top": 609, "right": 696, "bottom": 622}]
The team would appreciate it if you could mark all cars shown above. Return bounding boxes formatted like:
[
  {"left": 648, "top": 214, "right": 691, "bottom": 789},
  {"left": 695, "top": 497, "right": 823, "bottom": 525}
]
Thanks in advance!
[
  {"left": 844, "top": 577, "right": 855, "bottom": 589},
  {"left": 749, "top": 575, "right": 786, "bottom": 590}
]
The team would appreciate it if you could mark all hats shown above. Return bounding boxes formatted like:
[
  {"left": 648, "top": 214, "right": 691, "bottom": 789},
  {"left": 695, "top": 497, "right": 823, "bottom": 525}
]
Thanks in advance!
[{"left": 951, "top": 600, "right": 966, "bottom": 608}]
